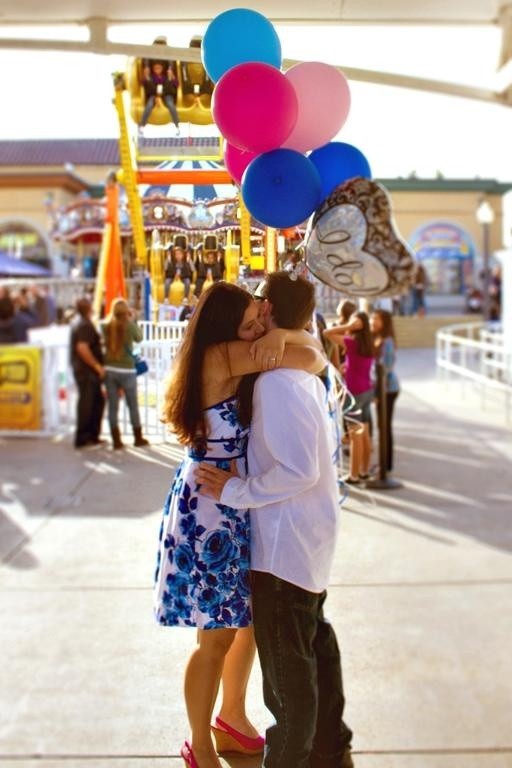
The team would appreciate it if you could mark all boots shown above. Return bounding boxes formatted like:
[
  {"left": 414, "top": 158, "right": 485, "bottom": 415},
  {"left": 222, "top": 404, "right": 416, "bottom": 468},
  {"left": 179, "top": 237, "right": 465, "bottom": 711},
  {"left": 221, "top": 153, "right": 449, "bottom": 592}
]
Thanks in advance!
[
  {"left": 133, "top": 425, "right": 149, "bottom": 447},
  {"left": 110, "top": 425, "right": 125, "bottom": 450}
]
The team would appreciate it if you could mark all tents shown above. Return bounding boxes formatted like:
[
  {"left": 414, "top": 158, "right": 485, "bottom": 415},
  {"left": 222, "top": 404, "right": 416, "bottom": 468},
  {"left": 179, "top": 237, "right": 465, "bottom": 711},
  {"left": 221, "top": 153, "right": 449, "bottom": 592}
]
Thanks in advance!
[{"left": 0, "top": 250, "right": 53, "bottom": 276}]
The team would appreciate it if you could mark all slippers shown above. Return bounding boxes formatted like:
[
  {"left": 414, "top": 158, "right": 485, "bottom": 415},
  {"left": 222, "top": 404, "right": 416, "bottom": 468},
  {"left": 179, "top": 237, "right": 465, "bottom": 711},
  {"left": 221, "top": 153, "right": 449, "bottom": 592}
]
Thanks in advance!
[
  {"left": 358, "top": 471, "right": 369, "bottom": 480},
  {"left": 343, "top": 475, "right": 361, "bottom": 484}
]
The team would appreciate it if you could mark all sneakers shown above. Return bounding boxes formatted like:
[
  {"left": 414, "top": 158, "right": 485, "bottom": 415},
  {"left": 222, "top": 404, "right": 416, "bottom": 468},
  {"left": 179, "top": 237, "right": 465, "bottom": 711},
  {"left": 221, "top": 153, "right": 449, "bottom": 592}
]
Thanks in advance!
[
  {"left": 74, "top": 438, "right": 107, "bottom": 449},
  {"left": 310, "top": 742, "right": 354, "bottom": 768}
]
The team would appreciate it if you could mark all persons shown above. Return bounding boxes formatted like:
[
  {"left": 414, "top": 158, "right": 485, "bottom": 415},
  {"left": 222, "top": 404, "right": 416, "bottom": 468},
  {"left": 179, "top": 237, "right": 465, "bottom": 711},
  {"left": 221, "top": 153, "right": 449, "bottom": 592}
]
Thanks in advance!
[
  {"left": 195, "top": 251, "right": 224, "bottom": 298},
  {"left": 194, "top": 270, "right": 355, "bottom": 767},
  {"left": 368, "top": 309, "right": 399, "bottom": 475},
  {"left": 0, "top": 283, "right": 55, "bottom": 345},
  {"left": 68, "top": 298, "right": 107, "bottom": 450},
  {"left": 323, "top": 312, "right": 375, "bottom": 488},
  {"left": 153, "top": 282, "right": 327, "bottom": 768},
  {"left": 323, "top": 301, "right": 375, "bottom": 457},
  {"left": 283, "top": 248, "right": 298, "bottom": 270},
  {"left": 138, "top": 62, "right": 183, "bottom": 136},
  {"left": 412, "top": 265, "right": 427, "bottom": 320},
  {"left": 163, "top": 246, "right": 192, "bottom": 297},
  {"left": 392, "top": 301, "right": 404, "bottom": 316},
  {"left": 305, "top": 313, "right": 343, "bottom": 492},
  {"left": 100, "top": 299, "right": 150, "bottom": 450}
]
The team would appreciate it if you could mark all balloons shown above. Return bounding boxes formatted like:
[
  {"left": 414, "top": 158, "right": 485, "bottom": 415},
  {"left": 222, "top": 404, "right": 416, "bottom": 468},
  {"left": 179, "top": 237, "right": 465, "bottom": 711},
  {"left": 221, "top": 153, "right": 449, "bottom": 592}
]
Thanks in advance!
[
  {"left": 240, "top": 148, "right": 322, "bottom": 230},
  {"left": 200, "top": 9, "right": 281, "bottom": 81},
  {"left": 290, "top": 176, "right": 418, "bottom": 302},
  {"left": 279, "top": 60, "right": 350, "bottom": 153},
  {"left": 210, "top": 61, "right": 299, "bottom": 148},
  {"left": 307, "top": 142, "right": 372, "bottom": 218},
  {"left": 223, "top": 141, "right": 257, "bottom": 183}
]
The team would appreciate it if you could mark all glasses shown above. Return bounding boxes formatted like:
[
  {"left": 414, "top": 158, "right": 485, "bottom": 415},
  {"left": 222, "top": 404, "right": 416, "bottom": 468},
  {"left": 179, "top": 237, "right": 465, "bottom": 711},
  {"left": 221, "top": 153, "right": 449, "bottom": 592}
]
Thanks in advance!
[{"left": 252, "top": 293, "right": 274, "bottom": 303}]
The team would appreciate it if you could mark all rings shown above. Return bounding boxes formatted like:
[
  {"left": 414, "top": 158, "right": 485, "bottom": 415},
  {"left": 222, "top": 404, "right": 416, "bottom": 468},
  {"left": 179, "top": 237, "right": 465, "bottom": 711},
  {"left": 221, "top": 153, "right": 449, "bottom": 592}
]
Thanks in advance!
[{"left": 267, "top": 356, "right": 277, "bottom": 362}]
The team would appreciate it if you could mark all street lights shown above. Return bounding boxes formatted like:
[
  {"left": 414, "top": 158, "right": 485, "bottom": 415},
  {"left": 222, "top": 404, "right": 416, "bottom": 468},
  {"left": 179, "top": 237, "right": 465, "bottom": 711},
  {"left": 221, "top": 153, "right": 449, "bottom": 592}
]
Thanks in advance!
[{"left": 475, "top": 200, "right": 493, "bottom": 320}]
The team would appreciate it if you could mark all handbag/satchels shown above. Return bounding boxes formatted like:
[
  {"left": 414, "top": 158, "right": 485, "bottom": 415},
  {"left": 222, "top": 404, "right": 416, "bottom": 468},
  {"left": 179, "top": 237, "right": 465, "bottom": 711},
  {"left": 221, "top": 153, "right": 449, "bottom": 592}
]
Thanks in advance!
[{"left": 124, "top": 342, "right": 149, "bottom": 375}]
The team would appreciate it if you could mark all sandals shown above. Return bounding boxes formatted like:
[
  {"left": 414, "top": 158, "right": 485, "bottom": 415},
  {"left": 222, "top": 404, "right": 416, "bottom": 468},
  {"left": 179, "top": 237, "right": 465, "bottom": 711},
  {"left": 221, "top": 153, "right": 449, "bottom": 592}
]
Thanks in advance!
[
  {"left": 210, "top": 716, "right": 265, "bottom": 757},
  {"left": 179, "top": 741, "right": 223, "bottom": 768}
]
